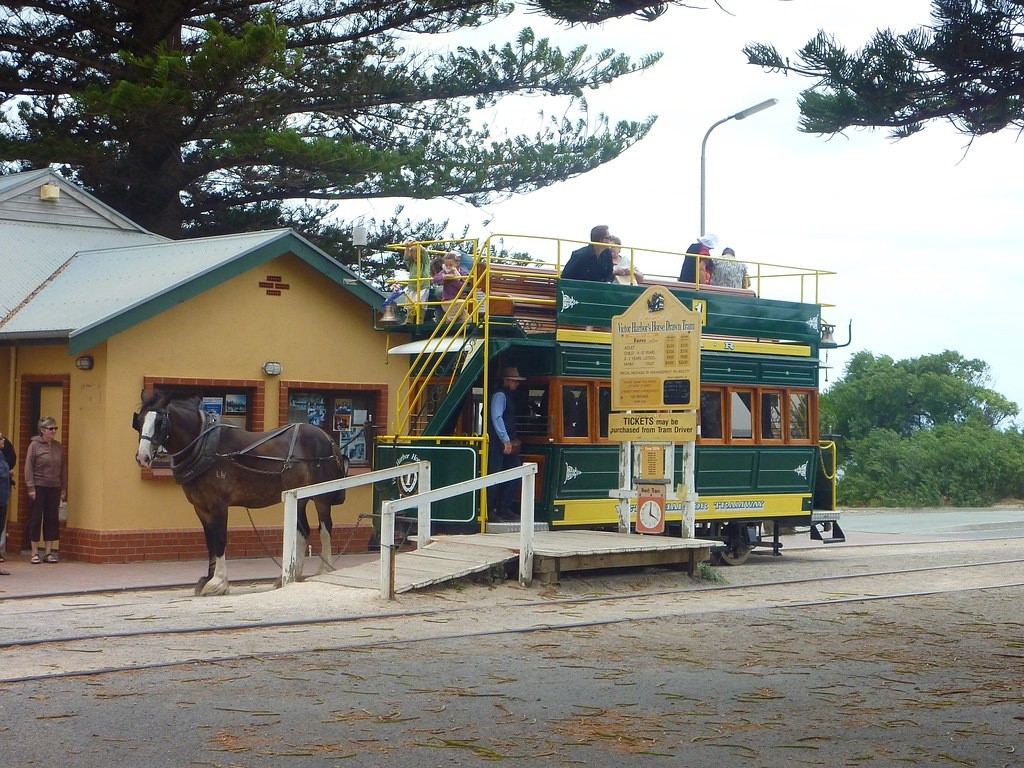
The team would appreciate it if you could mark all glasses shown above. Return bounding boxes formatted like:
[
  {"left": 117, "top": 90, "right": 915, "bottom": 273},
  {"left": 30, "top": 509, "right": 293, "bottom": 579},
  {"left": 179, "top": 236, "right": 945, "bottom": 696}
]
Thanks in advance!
[
  {"left": 40, "top": 425, "right": 59, "bottom": 432},
  {"left": 0, "top": 435, "right": 4, "bottom": 441},
  {"left": 601, "top": 236, "right": 612, "bottom": 242}
]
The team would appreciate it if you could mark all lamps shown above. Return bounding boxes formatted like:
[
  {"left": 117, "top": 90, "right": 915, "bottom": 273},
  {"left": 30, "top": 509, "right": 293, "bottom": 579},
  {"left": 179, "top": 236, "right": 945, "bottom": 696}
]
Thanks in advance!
[
  {"left": 262, "top": 361, "right": 282, "bottom": 375},
  {"left": 75, "top": 356, "right": 94, "bottom": 370}
]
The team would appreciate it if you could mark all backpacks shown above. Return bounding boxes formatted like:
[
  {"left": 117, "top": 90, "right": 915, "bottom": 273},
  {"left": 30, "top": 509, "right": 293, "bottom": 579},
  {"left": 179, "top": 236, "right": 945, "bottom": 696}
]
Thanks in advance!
[{"left": 1, "top": 437, "right": 17, "bottom": 470}]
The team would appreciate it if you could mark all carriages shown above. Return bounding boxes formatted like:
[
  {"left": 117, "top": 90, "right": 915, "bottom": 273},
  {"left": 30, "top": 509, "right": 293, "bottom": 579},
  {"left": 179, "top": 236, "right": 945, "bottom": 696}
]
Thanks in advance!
[{"left": 129, "top": 235, "right": 854, "bottom": 599}]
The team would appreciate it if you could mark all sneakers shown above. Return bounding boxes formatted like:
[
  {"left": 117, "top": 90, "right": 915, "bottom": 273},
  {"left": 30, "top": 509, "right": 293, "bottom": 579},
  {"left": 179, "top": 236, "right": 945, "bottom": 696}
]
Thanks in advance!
[
  {"left": 499, "top": 507, "right": 519, "bottom": 521},
  {"left": 483, "top": 508, "right": 502, "bottom": 525}
]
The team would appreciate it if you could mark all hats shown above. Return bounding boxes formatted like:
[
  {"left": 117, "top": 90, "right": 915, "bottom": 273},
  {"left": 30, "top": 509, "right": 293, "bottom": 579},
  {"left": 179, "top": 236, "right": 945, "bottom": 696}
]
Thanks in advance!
[
  {"left": 499, "top": 367, "right": 528, "bottom": 382},
  {"left": 697, "top": 233, "right": 719, "bottom": 250}
]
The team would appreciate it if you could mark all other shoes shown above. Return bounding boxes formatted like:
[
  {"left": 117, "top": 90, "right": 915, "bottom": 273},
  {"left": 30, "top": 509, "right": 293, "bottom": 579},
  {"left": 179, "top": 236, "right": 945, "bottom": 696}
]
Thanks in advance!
[
  {"left": 401, "top": 308, "right": 415, "bottom": 326},
  {"left": 0, "top": 566, "right": 11, "bottom": 575},
  {"left": 0, "top": 556, "right": 5, "bottom": 562},
  {"left": 416, "top": 306, "right": 427, "bottom": 326}
]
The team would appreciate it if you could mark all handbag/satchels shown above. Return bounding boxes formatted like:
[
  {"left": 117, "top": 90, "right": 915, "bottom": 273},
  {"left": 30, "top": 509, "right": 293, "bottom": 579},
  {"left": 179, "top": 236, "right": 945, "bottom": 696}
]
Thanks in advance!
[
  {"left": 612, "top": 269, "right": 638, "bottom": 287},
  {"left": 501, "top": 439, "right": 523, "bottom": 455}
]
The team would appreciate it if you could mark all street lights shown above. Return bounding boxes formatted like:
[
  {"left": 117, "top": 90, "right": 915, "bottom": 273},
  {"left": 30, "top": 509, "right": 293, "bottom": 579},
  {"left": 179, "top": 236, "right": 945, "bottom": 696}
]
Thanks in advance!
[{"left": 700, "top": 99, "right": 777, "bottom": 236}]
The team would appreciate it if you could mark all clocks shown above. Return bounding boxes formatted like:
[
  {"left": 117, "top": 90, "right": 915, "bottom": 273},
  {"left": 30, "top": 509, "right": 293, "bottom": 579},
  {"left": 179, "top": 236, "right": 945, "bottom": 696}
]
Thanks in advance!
[{"left": 635, "top": 496, "right": 666, "bottom": 534}]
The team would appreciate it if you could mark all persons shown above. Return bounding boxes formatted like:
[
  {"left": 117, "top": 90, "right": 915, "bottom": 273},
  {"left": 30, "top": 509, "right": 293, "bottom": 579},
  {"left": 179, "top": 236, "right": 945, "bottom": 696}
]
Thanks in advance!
[
  {"left": 680, "top": 234, "right": 714, "bottom": 286},
  {"left": 431, "top": 250, "right": 473, "bottom": 325},
  {"left": 608, "top": 234, "right": 644, "bottom": 288},
  {"left": 561, "top": 226, "right": 614, "bottom": 331},
  {"left": 403, "top": 240, "right": 432, "bottom": 325},
  {"left": 710, "top": 248, "right": 752, "bottom": 291},
  {"left": 25, "top": 416, "right": 68, "bottom": 565},
  {"left": 335, "top": 400, "right": 352, "bottom": 414},
  {"left": 0, "top": 431, "right": 17, "bottom": 576},
  {"left": 477, "top": 368, "right": 526, "bottom": 523}
]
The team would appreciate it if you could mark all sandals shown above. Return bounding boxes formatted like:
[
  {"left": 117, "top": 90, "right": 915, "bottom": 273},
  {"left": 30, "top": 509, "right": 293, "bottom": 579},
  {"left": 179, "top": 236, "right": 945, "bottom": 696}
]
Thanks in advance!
[
  {"left": 31, "top": 552, "right": 41, "bottom": 564},
  {"left": 43, "top": 553, "right": 59, "bottom": 564}
]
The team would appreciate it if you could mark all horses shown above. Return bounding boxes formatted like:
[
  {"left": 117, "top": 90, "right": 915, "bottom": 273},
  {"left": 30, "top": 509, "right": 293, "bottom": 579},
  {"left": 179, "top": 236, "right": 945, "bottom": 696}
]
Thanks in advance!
[{"left": 135, "top": 386, "right": 349, "bottom": 597}]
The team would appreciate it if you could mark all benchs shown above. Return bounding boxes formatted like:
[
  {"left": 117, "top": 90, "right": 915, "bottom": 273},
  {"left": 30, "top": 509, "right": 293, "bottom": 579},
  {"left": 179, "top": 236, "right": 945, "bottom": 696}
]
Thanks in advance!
[{"left": 477, "top": 264, "right": 757, "bottom": 316}]
